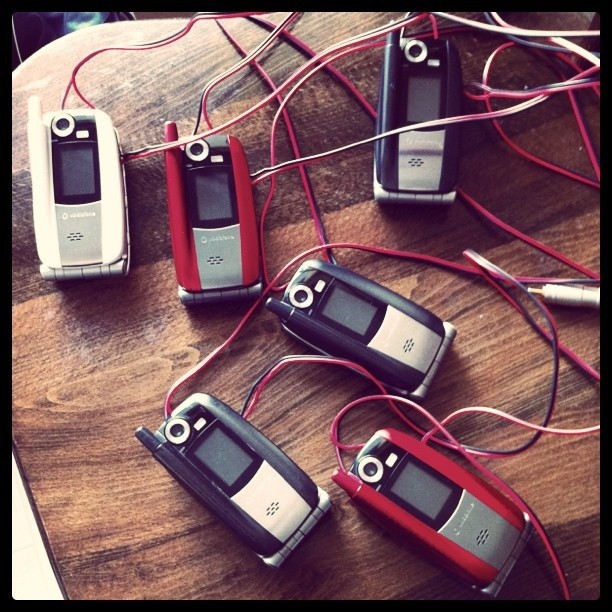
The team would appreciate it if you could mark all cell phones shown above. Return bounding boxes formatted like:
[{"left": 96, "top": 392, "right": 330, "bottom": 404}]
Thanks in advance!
[
  {"left": 162, "top": 120, "right": 265, "bottom": 306},
  {"left": 262, "top": 259, "right": 461, "bottom": 402},
  {"left": 374, "top": 31, "right": 463, "bottom": 205},
  {"left": 21, "top": 95, "right": 131, "bottom": 284},
  {"left": 330, "top": 426, "right": 532, "bottom": 595},
  {"left": 130, "top": 392, "right": 330, "bottom": 568}
]
